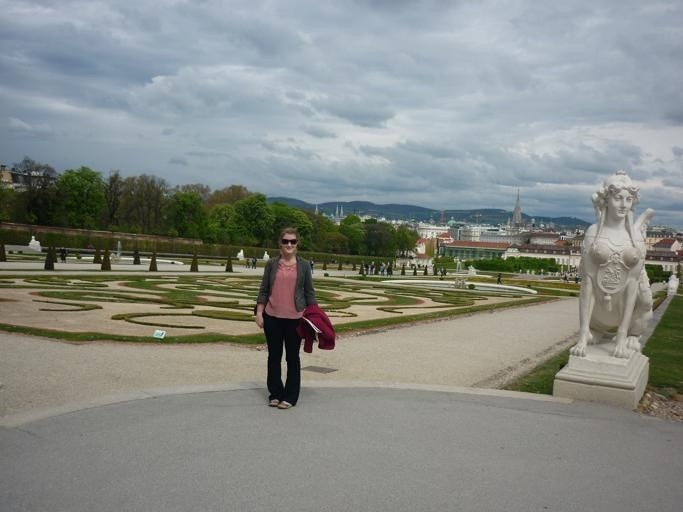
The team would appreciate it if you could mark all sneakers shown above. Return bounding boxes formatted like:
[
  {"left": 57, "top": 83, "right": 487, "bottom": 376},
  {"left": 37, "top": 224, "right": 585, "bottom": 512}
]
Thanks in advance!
[{"left": 269, "top": 400, "right": 292, "bottom": 409}]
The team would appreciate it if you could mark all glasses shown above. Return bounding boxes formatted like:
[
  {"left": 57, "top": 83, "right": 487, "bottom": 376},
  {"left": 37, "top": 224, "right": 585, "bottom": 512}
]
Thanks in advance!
[{"left": 281, "top": 238, "right": 297, "bottom": 245}]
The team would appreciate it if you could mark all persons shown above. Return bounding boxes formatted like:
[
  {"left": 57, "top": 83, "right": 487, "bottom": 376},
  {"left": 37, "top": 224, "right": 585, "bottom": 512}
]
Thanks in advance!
[
  {"left": 253, "top": 227, "right": 320, "bottom": 409},
  {"left": 363, "top": 261, "right": 393, "bottom": 277},
  {"left": 245, "top": 258, "right": 251, "bottom": 268},
  {"left": 497, "top": 273, "right": 503, "bottom": 284},
  {"left": 309, "top": 256, "right": 315, "bottom": 274},
  {"left": 569, "top": 169, "right": 655, "bottom": 359},
  {"left": 47, "top": 244, "right": 67, "bottom": 263},
  {"left": 251, "top": 255, "right": 258, "bottom": 269}
]
